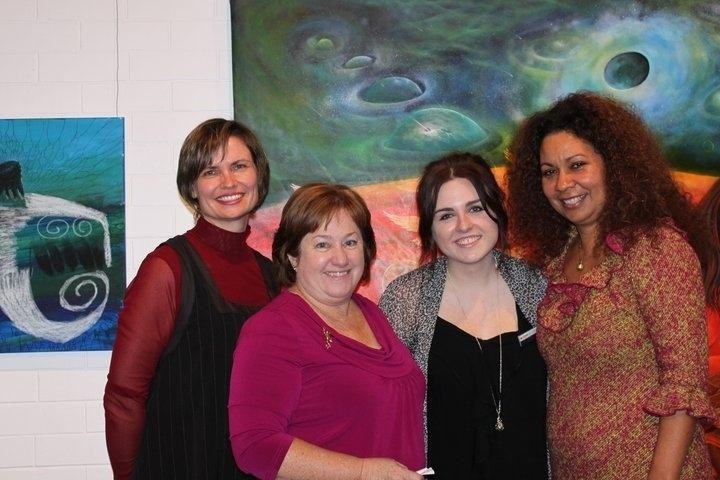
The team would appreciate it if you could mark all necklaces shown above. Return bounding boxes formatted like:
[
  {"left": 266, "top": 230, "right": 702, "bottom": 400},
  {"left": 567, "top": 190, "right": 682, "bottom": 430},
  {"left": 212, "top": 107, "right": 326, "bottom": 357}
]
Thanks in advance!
[
  {"left": 443, "top": 258, "right": 510, "bottom": 433},
  {"left": 575, "top": 239, "right": 595, "bottom": 275}
]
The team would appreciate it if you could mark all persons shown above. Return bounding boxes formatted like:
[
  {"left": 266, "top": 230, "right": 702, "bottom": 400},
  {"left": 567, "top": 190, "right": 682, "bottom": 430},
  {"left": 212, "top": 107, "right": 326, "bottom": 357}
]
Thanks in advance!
[
  {"left": 102, "top": 115, "right": 278, "bottom": 480},
  {"left": 226, "top": 175, "right": 433, "bottom": 479},
  {"left": 375, "top": 148, "right": 558, "bottom": 480},
  {"left": 506, "top": 90, "right": 719, "bottom": 479}
]
